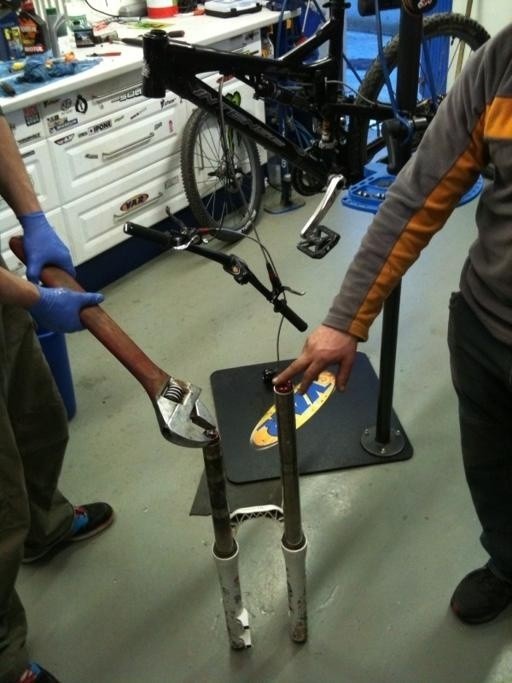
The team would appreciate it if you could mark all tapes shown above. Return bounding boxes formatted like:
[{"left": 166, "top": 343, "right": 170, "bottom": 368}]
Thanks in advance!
[{"left": 147, "top": 0, "right": 178, "bottom": 18}]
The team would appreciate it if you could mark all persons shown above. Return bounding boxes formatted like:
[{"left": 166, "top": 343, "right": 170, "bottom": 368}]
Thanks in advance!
[
  {"left": 272, "top": 23, "right": 512, "bottom": 625},
  {"left": 1, "top": 109, "right": 115, "bottom": 682}
]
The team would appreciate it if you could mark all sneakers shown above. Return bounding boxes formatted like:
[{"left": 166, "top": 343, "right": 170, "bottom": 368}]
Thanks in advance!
[
  {"left": 22, "top": 503, "right": 113, "bottom": 563},
  {"left": 18, "top": 661, "right": 60, "bottom": 683},
  {"left": 451, "top": 565, "right": 512, "bottom": 625}
]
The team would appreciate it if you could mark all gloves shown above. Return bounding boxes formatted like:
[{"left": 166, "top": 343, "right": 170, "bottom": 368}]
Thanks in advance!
[
  {"left": 16, "top": 211, "right": 76, "bottom": 285},
  {"left": 28, "top": 285, "right": 104, "bottom": 333}
]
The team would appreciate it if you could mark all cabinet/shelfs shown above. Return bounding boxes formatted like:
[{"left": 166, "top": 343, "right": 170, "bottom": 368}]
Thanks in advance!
[{"left": 0, "top": 8, "right": 301, "bottom": 284}]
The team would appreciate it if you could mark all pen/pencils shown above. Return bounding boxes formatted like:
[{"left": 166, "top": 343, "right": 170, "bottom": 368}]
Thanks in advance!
[{"left": 85, "top": 52, "right": 122, "bottom": 57}]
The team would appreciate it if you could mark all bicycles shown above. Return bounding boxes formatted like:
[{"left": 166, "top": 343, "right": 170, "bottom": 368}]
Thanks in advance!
[{"left": 122, "top": 0, "right": 491, "bottom": 332}]
